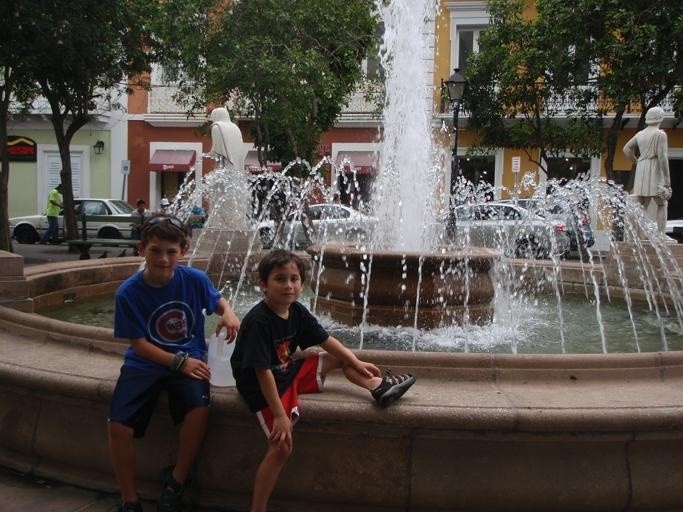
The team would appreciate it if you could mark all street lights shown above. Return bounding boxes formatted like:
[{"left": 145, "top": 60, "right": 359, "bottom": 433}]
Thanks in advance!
[{"left": 439, "top": 65, "right": 469, "bottom": 253}]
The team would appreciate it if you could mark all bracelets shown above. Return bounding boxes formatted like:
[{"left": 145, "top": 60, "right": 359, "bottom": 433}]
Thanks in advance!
[{"left": 172, "top": 352, "right": 189, "bottom": 371}]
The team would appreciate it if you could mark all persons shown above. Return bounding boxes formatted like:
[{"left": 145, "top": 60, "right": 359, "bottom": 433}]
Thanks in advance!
[
  {"left": 230, "top": 249, "right": 416, "bottom": 512},
  {"left": 35, "top": 184, "right": 64, "bottom": 244},
  {"left": 208, "top": 106, "right": 248, "bottom": 230},
  {"left": 624, "top": 106, "right": 674, "bottom": 241},
  {"left": 108, "top": 216, "right": 242, "bottom": 510},
  {"left": 129, "top": 198, "right": 206, "bottom": 242}
]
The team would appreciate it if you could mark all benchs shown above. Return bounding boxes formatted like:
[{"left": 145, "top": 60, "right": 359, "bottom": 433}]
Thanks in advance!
[{"left": 63, "top": 212, "right": 148, "bottom": 259}]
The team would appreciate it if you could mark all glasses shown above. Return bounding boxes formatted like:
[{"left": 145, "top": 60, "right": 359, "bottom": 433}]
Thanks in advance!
[{"left": 147, "top": 214, "right": 185, "bottom": 229}]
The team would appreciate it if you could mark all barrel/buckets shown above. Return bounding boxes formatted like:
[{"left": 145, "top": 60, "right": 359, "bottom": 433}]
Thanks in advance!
[{"left": 207, "top": 326, "right": 240, "bottom": 388}]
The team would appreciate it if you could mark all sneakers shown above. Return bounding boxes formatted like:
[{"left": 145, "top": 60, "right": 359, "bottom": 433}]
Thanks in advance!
[{"left": 370, "top": 371, "right": 417, "bottom": 407}]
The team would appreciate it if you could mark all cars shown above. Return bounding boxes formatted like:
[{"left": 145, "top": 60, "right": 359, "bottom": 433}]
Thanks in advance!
[
  {"left": 428, "top": 201, "right": 572, "bottom": 262},
  {"left": 7, "top": 196, "right": 136, "bottom": 248},
  {"left": 252, "top": 202, "right": 378, "bottom": 252}
]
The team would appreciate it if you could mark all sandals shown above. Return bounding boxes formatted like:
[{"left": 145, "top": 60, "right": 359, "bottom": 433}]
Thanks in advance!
[
  {"left": 158, "top": 464, "right": 190, "bottom": 512},
  {"left": 117, "top": 500, "right": 143, "bottom": 512}
]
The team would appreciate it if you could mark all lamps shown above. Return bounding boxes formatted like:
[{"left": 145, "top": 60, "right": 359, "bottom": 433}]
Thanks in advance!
[{"left": 94, "top": 139, "right": 104, "bottom": 155}]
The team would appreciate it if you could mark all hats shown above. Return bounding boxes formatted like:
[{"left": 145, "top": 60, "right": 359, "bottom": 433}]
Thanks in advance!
[{"left": 160, "top": 198, "right": 169, "bottom": 205}]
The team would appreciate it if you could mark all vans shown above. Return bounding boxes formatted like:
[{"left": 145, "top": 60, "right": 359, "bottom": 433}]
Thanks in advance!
[{"left": 485, "top": 196, "right": 595, "bottom": 253}]
